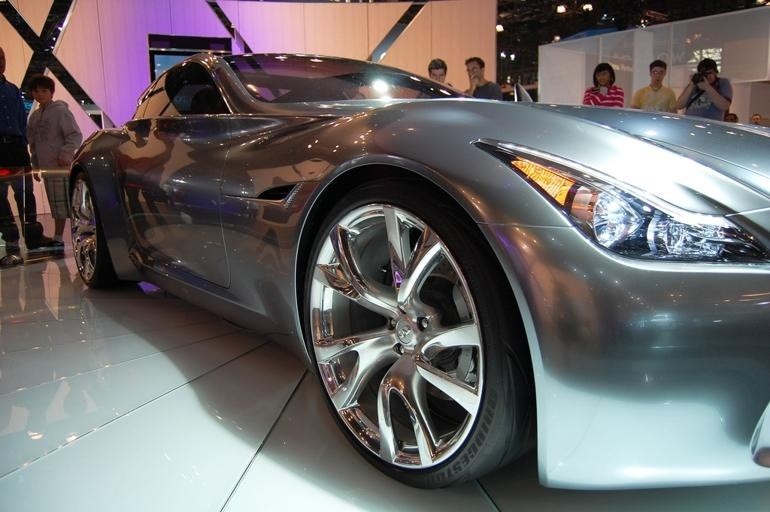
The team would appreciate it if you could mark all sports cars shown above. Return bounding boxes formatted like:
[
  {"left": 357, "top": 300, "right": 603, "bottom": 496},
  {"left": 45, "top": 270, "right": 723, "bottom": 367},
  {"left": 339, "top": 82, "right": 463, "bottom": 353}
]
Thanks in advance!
[{"left": 69, "top": 52, "right": 770, "bottom": 493}]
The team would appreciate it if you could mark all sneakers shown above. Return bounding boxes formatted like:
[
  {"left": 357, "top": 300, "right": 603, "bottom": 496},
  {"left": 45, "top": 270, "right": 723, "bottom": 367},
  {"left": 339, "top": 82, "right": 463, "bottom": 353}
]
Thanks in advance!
[{"left": 5, "top": 235, "right": 65, "bottom": 255}]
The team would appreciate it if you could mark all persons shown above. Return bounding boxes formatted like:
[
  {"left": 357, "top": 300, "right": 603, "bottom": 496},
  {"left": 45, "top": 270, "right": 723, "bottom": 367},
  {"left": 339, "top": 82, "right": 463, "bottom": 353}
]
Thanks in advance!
[
  {"left": 582, "top": 61, "right": 626, "bottom": 109},
  {"left": 26, "top": 73, "right": 84, "bottom": 255},
  {"left": 0, "top": 47, "right": 64, "bottom": 256},
  {"left": 726, "top": 112, "right": 738, "bottom": 122},
  {"left": 464, "top": 55, "right": 504, "bottom": 101},
  {"left": 629, "top": 59, "right": 678, "bottom": 114},
  {"left": 751, "top": 113, "right": 762, "bottom": 125},
  {"left": 675, "top": 57, "right": 734, "bottom": 122},
  {"left": 419, "top": 57, "right": 448, "bottom": 99}
]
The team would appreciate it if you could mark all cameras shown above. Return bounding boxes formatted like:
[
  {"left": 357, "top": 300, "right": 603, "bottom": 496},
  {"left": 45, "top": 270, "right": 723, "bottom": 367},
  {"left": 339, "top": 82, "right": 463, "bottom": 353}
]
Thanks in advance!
[{"left": 691, "top": 72, "right": 706, "bottom": 84}]
